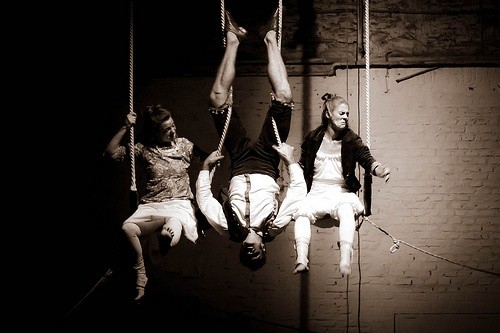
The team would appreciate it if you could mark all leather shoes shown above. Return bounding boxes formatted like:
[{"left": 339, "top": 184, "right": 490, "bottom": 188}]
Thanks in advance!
[
  {"left": 225, "top": 10, "right": 247, "bottom": 37},
  {"left": 254, "top": 5, "right": 283, "bottom": 41}
]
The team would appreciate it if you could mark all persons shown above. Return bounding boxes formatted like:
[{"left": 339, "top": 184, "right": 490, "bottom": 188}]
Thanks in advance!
[
  {"left": 196, "top": 7, "right": 308, "bottom": 272},
  {"left": 106, "top": 103, "right": 210, "bottom": 301},
  {"left": 292, "top": 93, "right": 390, "bottom": 276}
]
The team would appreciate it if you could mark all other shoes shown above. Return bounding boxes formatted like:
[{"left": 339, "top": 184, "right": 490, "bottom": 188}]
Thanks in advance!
[{"left": 133, "top": 257, "right": 148, "bottom": 300}]
